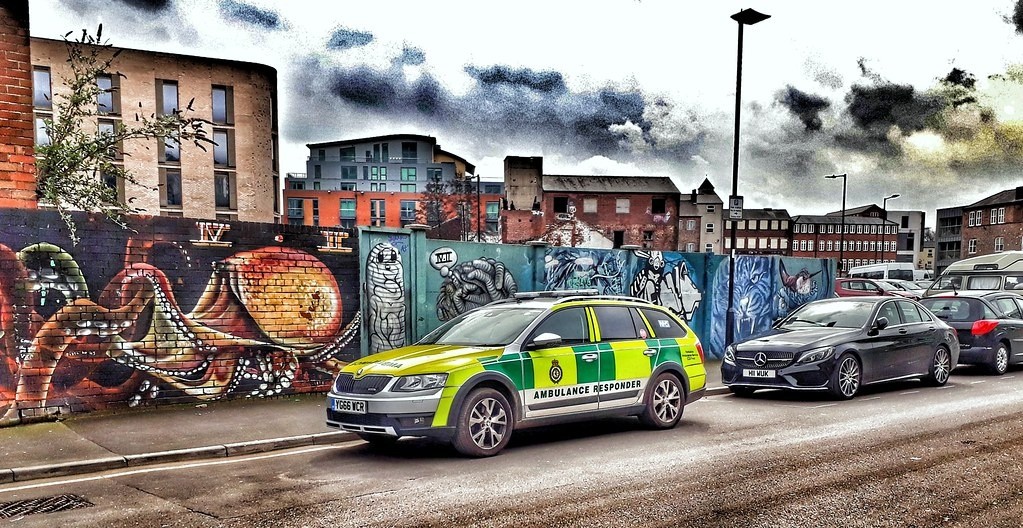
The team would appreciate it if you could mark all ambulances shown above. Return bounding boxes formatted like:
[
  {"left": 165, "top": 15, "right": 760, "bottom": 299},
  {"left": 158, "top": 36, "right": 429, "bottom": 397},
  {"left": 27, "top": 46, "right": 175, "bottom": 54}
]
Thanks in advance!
[{"left": 326, "top": 289, "right": 707, "bottom": 457}]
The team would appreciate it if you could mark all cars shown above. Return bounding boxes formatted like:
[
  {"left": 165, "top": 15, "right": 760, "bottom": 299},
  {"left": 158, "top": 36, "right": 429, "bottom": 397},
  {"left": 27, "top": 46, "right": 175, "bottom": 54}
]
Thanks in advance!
[
  {"left": 919, "top": 291, "right": 1023, "bottom": 375},
  {"left": 835, "top": 278, "right": 939, "bottom": 301},
  {"left": 721, "top": 296, "right": 960, "bottom": 400}
]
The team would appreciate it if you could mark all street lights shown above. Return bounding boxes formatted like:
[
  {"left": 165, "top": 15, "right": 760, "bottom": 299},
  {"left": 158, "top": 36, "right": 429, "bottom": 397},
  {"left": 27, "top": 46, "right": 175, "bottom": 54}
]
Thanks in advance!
[
  {"left": 726, "top": 8, "right": 772, "bottom": 355},
  {"left": 825, "top": 174, "right": 846, "bottom": 278},
  {"left": 882, "top": 195, "right": 901, "bottom": 263}
]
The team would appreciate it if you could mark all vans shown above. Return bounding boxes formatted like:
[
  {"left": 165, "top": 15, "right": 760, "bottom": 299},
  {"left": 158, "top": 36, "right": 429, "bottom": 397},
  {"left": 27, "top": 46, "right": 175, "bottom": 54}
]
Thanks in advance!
[
  {"left": 847, "top": 262, "right": 934, "bottom": 282},
  {"left": 922, "top": 251, "right": 1023, "bottom": 295}
]
